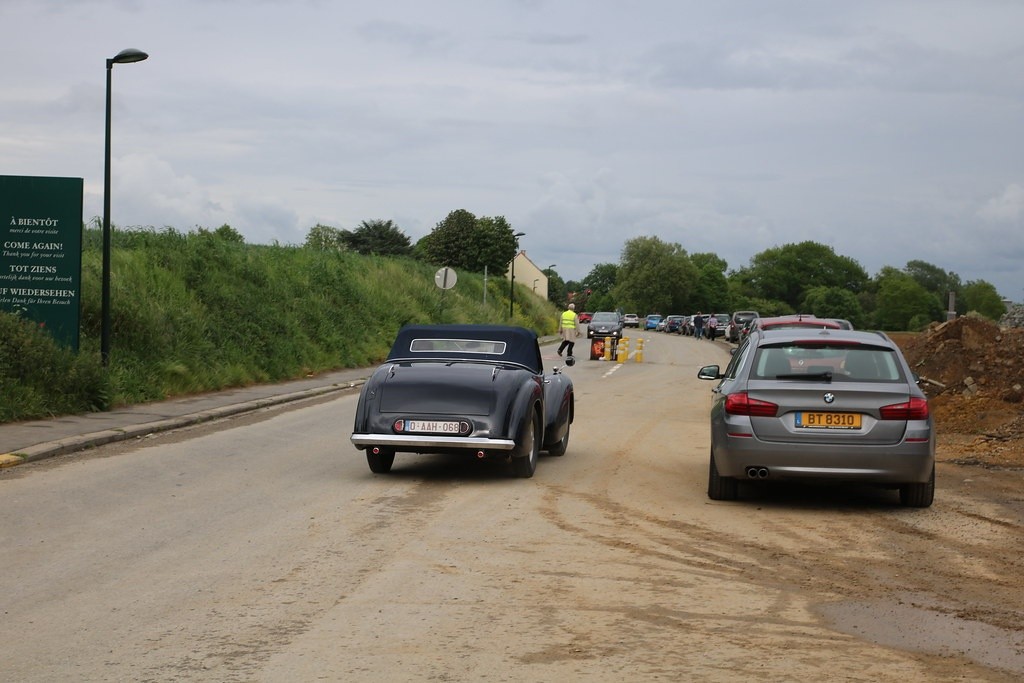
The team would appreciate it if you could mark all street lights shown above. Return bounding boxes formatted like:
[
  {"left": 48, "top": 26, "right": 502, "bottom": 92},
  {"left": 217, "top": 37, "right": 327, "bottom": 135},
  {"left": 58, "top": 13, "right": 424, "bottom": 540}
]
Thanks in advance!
[
  {"left": 547, "top": 264, "right": 557, "bottom": 300},
  {"left": 97, "top": 46, "right": 149, "bottom": 415},
  {"left": 510, "top": 232, "right": 526, "bottom": 318}
]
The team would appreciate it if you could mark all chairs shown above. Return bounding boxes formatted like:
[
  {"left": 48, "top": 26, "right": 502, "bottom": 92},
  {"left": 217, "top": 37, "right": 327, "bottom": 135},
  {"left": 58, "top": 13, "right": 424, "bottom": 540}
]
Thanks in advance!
[
  {"left": 844, "top": 352, "right": 858, "bottom": 374},
  {"left": 768, "top": 358, "right": 790, "bottom": 376}
]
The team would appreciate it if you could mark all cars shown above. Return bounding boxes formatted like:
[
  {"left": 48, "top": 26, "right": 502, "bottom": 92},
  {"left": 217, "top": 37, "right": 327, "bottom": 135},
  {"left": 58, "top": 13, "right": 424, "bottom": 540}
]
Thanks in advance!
[
  {"left": 349, "top": 323, "right": 577, "bottom": 479},
  {"left": 586, "top": 311, "right": 623, "bottom": 339},
  {"left": 653, "top": 310, "right": 854, "bottom": 376},
  {"left": 577, "top": 311, "right": 625, "bottom": 330},
  {"left": 697, "top": 327, "right": 938, "bottom": 509},
  {"left": 643, "top": 314, "right": 662, "bottom": 331},
  {"left": 623, "top": 313, "right": 639, "bottom": 329}
]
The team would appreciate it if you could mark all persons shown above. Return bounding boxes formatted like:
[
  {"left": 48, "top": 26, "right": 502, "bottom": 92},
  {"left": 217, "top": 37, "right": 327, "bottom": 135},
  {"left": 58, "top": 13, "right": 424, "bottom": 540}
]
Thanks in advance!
[
  {"left": 694, "top": 312, "right": 706, "bottom": 340},
  {"left": 706, "top": 313, "right": 718, "bottom": 341},
  {"left": 557, "top": 303, "right": 581, "bottom": 359}
]
